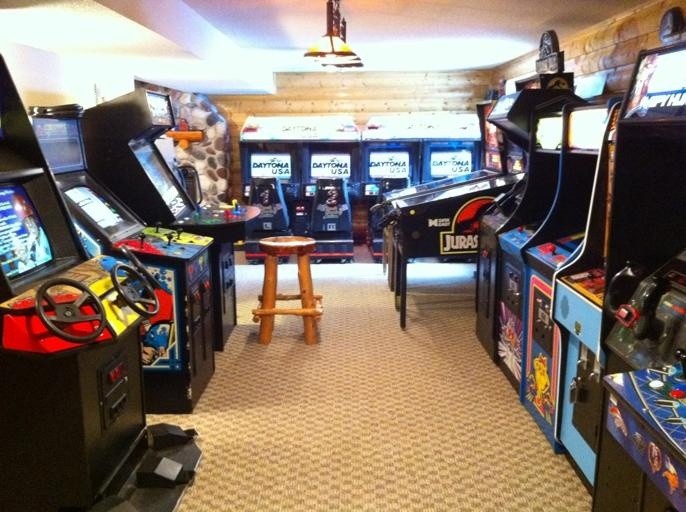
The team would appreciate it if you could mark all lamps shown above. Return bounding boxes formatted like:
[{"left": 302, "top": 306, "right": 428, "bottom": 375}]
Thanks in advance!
[{"left": 303, "top": 0, "right": 362, "bottom": 72}]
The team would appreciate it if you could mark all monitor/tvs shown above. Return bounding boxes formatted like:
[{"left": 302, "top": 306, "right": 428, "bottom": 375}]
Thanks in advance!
[
  {"left": 368, "top": 148, "right": 411, "bottom": 182},
  {"left": 0, "top": 184, "right": 55, "bottom": 283},
  {"left": 56, "top": 170, "right": 147, "bottom": 244},
  {"left": 248, "top": 149, "right": 293, "bottom": 184},
  {"left": 129, "top": 139, "right": 198, "bottom": 219},
  {"left": 309, "top": 149, "right": 353, "bottom": 183},
  {"left": 429, "top": 147, "right": 473, "bottom": 179}
]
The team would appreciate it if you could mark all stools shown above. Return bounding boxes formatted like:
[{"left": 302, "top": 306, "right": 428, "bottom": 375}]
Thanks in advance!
[{"left": 251, "top": 235, "right": 322, "bottom": 344}]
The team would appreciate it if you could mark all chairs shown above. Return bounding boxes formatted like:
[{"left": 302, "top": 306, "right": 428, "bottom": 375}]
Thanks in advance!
[
  {"left": 368, "top": 176, "right": 415, "bottom": 266},
  {"left": 239, "top": 175, "right": 294, "bottom": 266},
  {"left": 306, "top": 173, "right": 359, "bottom": 264}
]
